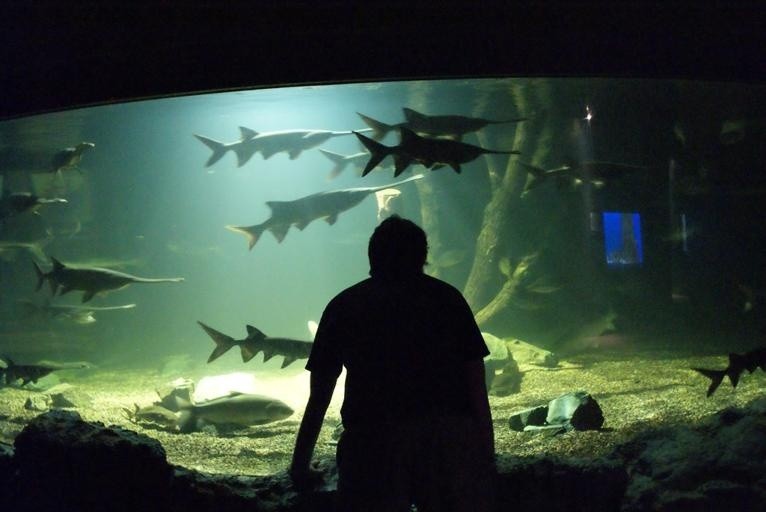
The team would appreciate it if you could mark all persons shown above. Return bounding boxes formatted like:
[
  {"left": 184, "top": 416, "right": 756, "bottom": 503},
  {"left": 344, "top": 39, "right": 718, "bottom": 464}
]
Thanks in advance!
[{"left": 291, "top": 214, "right": 495, "bottom": 512}]
